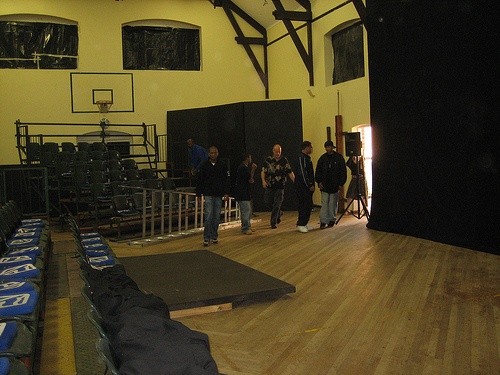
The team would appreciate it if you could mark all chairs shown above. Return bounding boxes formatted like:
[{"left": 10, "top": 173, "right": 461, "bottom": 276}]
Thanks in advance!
[
  {"left": 27, "top": 142, "right": 194, "bottom": 236},
  {"left": 0, "top": 200, "right": 51, "bottom": 375},
  {"left": 67, "top": 217, "right": 219, "bottom": 375}
]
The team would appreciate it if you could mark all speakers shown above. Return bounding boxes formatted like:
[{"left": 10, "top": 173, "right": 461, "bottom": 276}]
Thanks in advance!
[{"left": 345, "top": 132, "right": 361, "bottom": 156}]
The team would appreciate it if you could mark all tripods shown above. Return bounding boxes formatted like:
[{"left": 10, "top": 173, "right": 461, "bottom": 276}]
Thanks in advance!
[{"left": 335, "top": 156, "right": 370, "bottom": 225}]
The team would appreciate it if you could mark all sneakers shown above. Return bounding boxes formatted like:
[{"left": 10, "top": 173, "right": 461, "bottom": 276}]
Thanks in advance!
[{"left": 297, "top": 224, "right": 313, "bottom": 233}]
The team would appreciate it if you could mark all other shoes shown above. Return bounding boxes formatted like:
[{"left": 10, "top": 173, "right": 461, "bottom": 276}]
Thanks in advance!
[
  {"left": 210, "top": 239, "right": 218, "bottom": 244},
  {"left": 345, "top": 210, "right": 351, "bottom": 215},
  {"left": 328, "top": 221, "right": 335, "bottom": 228},
  {"left": 272, "top": 225, "right": 277, "bottom": 229},
  {"left": 243, "top": 227, "right": 256, "bottom": 235},
  {"left": 203, "top": 239, "right": 210, "bottom": 246},
  {"left": 277, "top": 209, "right": 283, "bottom": 224},
  {"left": 320, "top": 223, "right": 327, "bottom": 229}
]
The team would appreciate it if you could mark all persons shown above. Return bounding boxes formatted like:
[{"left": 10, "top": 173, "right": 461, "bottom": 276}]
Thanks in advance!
[
  {"left": 346, "top": 155, "right": 368, "bottom": 214},
  {"left": 314, "top": 139, "right": 347, "bottom": 229},
  {"left": 186, "top": 137, "right": 207, "bottom": 186},
  {"left": 233, "top": 152, "right": 258, "bottom": 235},
  {"left": 294, "top": 140, "right": 316, "bottom": 233},
  {"left": 165, "top": 159, "right": 181, "bottom": 184},
  {"left": 195, "top": 146, "right": 231, "bottom": 246},
  {"left": 260, "top": 144, "right": 296, "bottom": 230}
]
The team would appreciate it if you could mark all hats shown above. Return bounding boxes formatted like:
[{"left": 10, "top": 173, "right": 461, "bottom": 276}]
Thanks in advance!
[{"left": 324, "top": 141, "right": 337, "bottom": 149}]
